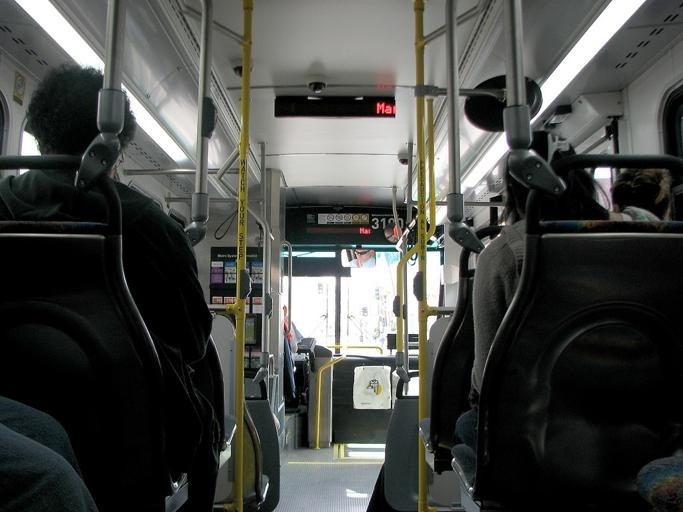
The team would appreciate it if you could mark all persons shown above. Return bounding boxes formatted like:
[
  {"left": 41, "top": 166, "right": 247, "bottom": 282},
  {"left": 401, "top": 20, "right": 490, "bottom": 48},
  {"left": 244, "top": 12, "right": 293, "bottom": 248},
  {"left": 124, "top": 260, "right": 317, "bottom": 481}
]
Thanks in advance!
[
  {"left": 283, "top": 306, "right": 298, "bottom": 359},
  {"left": 456, "top": 131, "right": 662, "bottom": 456},
  {"left": 609, "top": 165, "right": 672, "bottom": 222},
  {"left": 341, "top": 248, "right": 375, "bottom": 267},
  {"left": 0, "top": 395, "right": 97, "bottom": 512},
  {"left": 0, "top": 66, "right": 215, "bottom": 512}
]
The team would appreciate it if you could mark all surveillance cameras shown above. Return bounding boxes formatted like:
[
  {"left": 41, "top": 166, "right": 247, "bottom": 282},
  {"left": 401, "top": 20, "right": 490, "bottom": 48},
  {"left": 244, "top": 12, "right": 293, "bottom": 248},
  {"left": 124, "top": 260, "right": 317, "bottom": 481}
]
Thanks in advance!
[
  {"left": 398, "top": 149, "right": 412, "bottom": 166},
  {"left": 231, "top": 57, "right": 255, "bottom": 78},
  {"left": 306, "top": 75, "right": 328, "bottom": 95}
]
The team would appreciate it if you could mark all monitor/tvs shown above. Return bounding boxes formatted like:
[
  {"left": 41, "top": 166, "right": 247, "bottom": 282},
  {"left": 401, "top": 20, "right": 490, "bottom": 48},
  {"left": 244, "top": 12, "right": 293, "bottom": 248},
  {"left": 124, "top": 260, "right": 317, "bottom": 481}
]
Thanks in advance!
[{"left": 209, "top": 246, "right": 270, "bottom": 314}]
